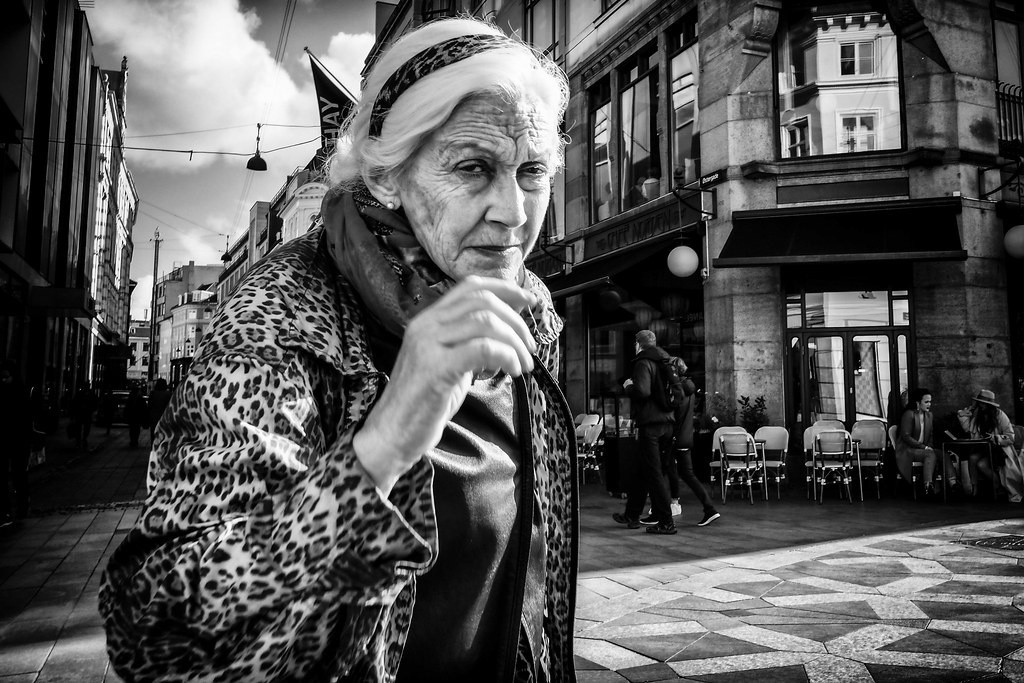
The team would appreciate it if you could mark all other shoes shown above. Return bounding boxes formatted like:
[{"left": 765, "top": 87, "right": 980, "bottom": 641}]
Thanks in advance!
[
  {"left": 922, "top": 484, "right": 936, "bottom": 499},
  {"left": 952, "top": 483, "right": 962, "bottom": 495}
]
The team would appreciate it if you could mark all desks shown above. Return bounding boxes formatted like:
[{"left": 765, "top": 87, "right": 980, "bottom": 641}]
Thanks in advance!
[
  {"left": 852, "top": 438, "right": 864, "bottom": 502},
  {"left": 942, "top": 441, "right": 996, "bottom": 506},
  {"left": 754, "top": 439, "right": 770, "bottom": 501}
]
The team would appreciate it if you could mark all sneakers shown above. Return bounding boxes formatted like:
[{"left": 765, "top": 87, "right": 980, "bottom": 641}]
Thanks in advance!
[
  {"left": 639, "top": 514, "right": 658, "bottom": 524},
  {"left": 698, "top": 508, "right": 720, "bottom": 526},
  {"left": 612, "top": 513, "right": 641, "bottom": 529},
  {"left": 649, "top": 502, "right": 682, "bottom": 517},
  {"left": 646, "top": 522, "right": 677, "bottom": 535}
]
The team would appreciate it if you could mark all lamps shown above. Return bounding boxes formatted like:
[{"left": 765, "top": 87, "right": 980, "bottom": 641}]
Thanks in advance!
[
  {"left": 667, "top": 183, "right": 717, "bottom": 277},
  {"left": 978, "top": 156, "right": 1024, "bottom": 259}
]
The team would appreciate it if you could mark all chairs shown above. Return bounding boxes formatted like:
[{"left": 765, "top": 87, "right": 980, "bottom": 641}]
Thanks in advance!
[
  {"left": 574, "top": 413, "right": 603, "bottom": 487},
  {"left": 803, "top": 419, "right": 923, "bottom": 504},
  {"left": 709, "top": 426, "right": 789, "bottom": 505},
  {"left": 600, "top": 412, "right": 631, "bottom": 436}
]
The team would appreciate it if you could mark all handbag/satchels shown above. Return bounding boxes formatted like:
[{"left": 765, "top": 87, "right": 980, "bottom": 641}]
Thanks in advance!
[{"left": 67, "top": 422, "right": 76, "bottom": 439}]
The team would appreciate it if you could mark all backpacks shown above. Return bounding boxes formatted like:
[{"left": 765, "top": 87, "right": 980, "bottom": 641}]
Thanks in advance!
[{"left": 654, "top": 359, "right": 685, "bottom": 411}]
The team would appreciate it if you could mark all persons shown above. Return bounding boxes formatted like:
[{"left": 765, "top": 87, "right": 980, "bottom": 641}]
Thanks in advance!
[
  {"left": 895, "top": 387, "right": 1024, "bottom": 506},
  {"left": 0, "top": 16, "right": 581, "bottom": 683},
  {"left": 612, "top": 330, "right": 721, "bottom": 534}
]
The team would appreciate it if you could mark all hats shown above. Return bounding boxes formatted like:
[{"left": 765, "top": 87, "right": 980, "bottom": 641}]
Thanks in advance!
[{"left": 972, "top": 389, "right": 1000, "bottom": 409}]
